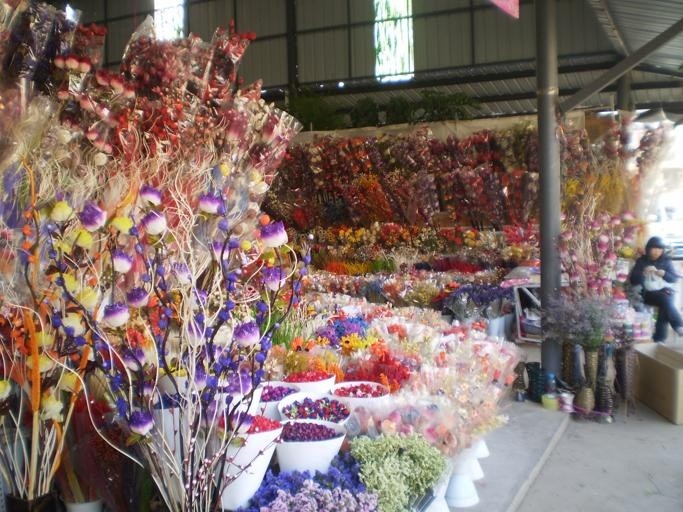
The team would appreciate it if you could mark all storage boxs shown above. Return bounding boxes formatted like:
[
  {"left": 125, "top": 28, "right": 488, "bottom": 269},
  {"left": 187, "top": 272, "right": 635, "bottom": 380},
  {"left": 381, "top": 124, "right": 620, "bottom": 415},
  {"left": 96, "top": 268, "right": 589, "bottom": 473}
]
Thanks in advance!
[{"left": 627, "top": 343, "right": 682, "bottom": 426}]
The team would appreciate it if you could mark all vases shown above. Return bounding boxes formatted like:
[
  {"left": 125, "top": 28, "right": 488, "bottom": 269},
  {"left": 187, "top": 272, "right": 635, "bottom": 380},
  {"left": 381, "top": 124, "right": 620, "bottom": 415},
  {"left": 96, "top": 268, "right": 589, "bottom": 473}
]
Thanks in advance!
[
  {"left": 601, "top": 345, "right": 617, "bottom": 394},
  {"left": 594, "top": 388, "right": 615, "bottom": 418},
  {"left": 587, "top": 346, "right": 599, "bottom": 387},
  {"left": 576, "top": 387, "right": 595, "bottom": 413},
  {"left": 542, "top": 394, "right": 561, "bottom": 410},
  {"left": 559, "top": 392, "right": 576, "bottom": 412},
  {"left": 574, "top": 344, "right": 586, "bottom": 386}
]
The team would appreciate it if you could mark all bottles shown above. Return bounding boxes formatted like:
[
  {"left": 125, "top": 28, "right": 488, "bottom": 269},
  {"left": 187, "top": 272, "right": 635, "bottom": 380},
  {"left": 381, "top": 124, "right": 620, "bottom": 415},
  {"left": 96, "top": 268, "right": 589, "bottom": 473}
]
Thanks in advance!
[{"left": 545, "top": 372, "right": 556, "bottom": 394}]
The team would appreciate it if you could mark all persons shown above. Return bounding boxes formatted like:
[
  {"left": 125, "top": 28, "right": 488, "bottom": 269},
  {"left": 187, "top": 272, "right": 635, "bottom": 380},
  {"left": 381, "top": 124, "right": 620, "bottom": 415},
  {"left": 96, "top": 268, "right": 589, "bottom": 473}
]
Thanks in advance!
[{"left": 631, "top": 237, "right": 683, "bottom": 342}]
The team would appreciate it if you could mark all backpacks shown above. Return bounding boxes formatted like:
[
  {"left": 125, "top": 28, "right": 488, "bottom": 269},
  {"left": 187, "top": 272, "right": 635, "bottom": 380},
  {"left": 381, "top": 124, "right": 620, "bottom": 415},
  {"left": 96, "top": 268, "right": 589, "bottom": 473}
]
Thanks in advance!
[{"left": 642, "top": 264, "right": 667, "bottom": 294}]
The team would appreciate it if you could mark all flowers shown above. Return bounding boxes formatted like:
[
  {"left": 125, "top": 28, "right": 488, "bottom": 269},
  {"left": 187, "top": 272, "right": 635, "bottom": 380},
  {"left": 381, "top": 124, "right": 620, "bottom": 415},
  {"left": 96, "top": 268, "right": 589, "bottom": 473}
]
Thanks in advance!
[
  {"left": 544, "top": 294, "right": 628, "bottom": 351},
  {"left": 276, "top": 291, "right": 518, "bottom": 510},
  {"left": 2, "top": 1, "right": 301, "bottom": 511},
  {"left": 259, "top": 108, "right": 669, "bottom": 320}
]
